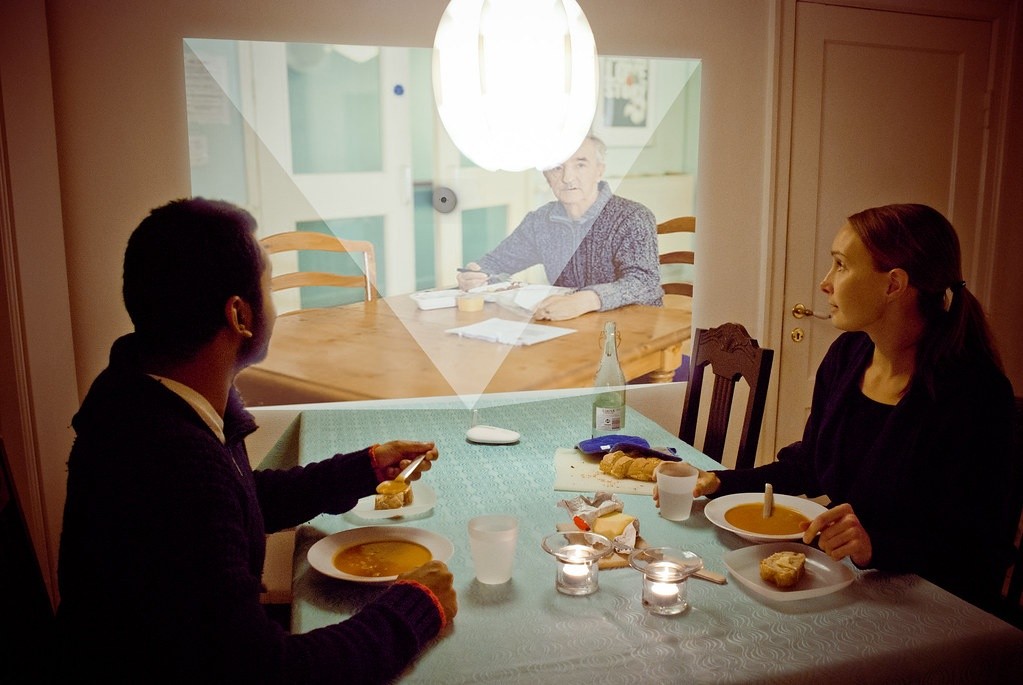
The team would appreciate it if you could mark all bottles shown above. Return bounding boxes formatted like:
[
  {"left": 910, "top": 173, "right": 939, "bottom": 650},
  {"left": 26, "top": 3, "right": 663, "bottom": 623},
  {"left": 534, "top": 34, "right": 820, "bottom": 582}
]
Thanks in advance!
[{"left": 592, "top": 321, "right": 629, "bottom": 433}]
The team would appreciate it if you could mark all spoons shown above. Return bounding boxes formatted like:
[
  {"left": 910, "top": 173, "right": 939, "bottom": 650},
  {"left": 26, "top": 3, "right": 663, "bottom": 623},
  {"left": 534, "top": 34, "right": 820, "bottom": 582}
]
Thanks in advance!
[{"left": 375, "top": 453, "right": 425, "bottom": 495}]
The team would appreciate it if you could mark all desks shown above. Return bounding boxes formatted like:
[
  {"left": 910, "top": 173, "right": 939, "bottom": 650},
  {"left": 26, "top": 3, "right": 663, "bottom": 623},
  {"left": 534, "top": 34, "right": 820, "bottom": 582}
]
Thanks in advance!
[
  {"left": 235, "top": 283, "right": 691, "bottom": 408},
  {"left": 255, "top": 393, "right": 1023, "bottom": 685}
]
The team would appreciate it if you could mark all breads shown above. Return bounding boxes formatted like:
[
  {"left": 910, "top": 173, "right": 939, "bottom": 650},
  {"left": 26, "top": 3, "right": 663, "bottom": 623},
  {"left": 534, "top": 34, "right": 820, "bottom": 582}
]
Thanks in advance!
[
  {"left": 599, "top": 450, "right": 664, "bottom": 481},
  {"left": 374, "top": 482, "right": 414, "bottom": 510},
  {"left": 759, "top": 550, "right": 806, "bottom": 587}
]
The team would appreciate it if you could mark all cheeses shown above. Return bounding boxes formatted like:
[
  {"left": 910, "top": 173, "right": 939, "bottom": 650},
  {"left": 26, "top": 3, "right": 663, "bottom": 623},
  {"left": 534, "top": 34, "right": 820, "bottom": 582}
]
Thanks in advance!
[{"left": 592, "top": 511, "right": 635, "bottom": 539}]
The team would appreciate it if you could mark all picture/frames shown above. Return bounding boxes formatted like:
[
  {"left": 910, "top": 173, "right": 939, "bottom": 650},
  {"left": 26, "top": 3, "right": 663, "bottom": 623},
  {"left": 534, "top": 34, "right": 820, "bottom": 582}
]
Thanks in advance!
[{"left": 595, "top": 55, "right": 654, "bottom": 145}]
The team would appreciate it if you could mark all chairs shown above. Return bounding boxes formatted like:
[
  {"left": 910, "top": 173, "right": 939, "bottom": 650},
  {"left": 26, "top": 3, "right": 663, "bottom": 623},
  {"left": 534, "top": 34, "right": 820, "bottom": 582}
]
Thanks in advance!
[
  {"left": 679, "top": 321, "right": 775, "bottom": 470},
  {"left": 655, "top": 215, "right": 698, "bottom": 303},
  {"left": 258, "top": 231, "right": 377, "bottom": 315}
]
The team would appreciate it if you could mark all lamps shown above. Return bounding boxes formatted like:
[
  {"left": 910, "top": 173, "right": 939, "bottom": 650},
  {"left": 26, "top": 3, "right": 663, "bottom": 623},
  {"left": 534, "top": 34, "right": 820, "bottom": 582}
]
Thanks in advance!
[{"left": 432, "top": 0, "right": 600, "bottom": 172}]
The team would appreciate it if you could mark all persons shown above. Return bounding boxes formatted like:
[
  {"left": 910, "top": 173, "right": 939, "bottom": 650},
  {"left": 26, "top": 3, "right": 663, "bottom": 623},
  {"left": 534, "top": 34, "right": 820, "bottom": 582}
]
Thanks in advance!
[
  {"left": 59, "top": 200, "right": 456, "bottom": 685},
  {"left": 653, "top": 199, "right": 1023, "bottom": 612},
  {"left": 456, "top": 136, "right": 663, "bottom": 323}
]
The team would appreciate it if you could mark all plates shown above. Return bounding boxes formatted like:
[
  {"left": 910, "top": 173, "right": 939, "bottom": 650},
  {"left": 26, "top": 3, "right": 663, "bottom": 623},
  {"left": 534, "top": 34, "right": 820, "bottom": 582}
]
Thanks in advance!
[
  {"left": 351, "top": 475, "right": 441, "bottom": 520},
  {"left": 306, "top": 525, "right": 456, "bottom": 583},
  {"left": 718, "top": 541, "right": 855, "bottom": 603},
  {"left": 704, "top": 492, "right": 829, "bottom": 544}
]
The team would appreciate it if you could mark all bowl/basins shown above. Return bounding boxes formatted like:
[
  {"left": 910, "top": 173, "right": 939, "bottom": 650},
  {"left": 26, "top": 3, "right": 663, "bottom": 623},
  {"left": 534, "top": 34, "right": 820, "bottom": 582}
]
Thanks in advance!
[
  {"left": 467, "top": 281, "right": 528, "bottom": 303},
  {"left": 408, "top": 288, "right": 466, "bottom": 311},
  {"left": 456, "top": 292, "right": 485, "bottom": 312}
]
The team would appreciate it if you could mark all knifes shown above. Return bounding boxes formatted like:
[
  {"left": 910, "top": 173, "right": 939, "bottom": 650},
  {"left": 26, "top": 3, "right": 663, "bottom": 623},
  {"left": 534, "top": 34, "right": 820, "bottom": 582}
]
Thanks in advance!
[{"left": 613, "top": 542, "right": 725, "bottom": 584}]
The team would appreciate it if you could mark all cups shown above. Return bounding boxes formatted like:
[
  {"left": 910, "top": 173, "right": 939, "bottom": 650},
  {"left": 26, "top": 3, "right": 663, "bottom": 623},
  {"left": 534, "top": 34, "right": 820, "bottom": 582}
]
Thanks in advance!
[
  {"left": 655, "top": 462, "right": 699, "bottom": 521},
  {"left": 467, "top": 514, "right": 519, "bottom": 585}
]
